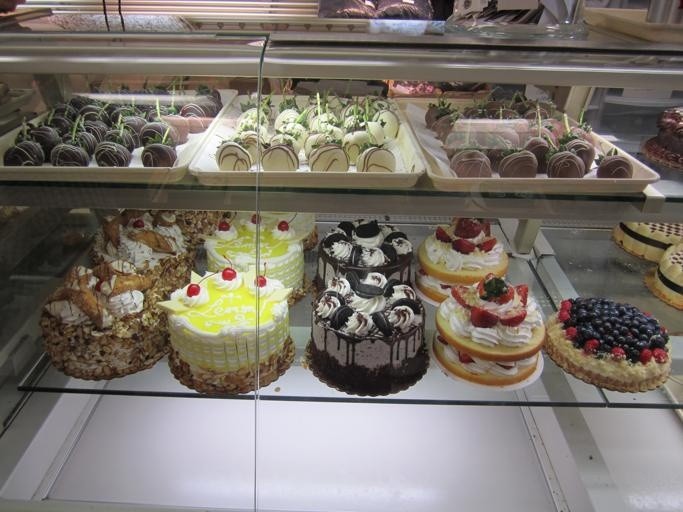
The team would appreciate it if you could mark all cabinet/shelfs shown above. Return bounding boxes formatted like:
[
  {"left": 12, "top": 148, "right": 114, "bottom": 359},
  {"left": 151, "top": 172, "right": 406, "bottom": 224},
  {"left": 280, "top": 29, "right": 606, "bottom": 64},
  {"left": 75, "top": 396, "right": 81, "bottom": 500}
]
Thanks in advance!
[{"left": 0, "top": 131, "right": 682, "bottom": 409}]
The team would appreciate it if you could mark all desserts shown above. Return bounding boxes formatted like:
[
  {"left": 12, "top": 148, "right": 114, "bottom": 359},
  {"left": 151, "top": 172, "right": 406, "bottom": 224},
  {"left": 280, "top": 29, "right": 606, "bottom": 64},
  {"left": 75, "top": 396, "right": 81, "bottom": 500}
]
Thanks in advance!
[
  {"left": 0, "top": 56, "right": 223, "bottom": 189},
  {"left": 214, "top": 88, "right": 399, "bottom": 175},
  {"left": 425, "top": 87, "right": 634, "bottom": 179}
]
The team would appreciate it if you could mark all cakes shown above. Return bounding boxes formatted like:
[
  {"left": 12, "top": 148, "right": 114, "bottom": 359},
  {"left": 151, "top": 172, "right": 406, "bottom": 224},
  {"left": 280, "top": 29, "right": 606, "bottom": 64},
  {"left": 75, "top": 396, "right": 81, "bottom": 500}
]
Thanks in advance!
[
  {"left": 202, "top": 218, "right": 308, "bottom": 307},
  {"left": 168, "top": 265, "right": 295, "bottom": 395},
  {"left": 642, "top": 101, "right": 683, "bottom": 172},
  {"left": 39, "top": 265, "right": 170, "bottom": 380},
  {"left": 431, "top": 273, "right": 546, "bottom": 385},
  {"left": 610, "top": 220, "right": 683, "bottom": 262},
  {"left": 90, "top": 205, "right": 220, "bottom": 291},
  {"left": 545, "top": 296, "right": 673, "bottom": 393},
  {"left": 654, "top": 241, "right": 683, "bottom": 309},
  {"left": 318, "top": 218, "right": 415, "bottom": 300},
  {"left": 236, "top": 211, "right": 320, "bottom": 252},
  {"left": 415, "top": 217, "right": 509, "bottom": 304},
  {"left": 309, "top": 270, "right": 426, "bottom": 389}
]
